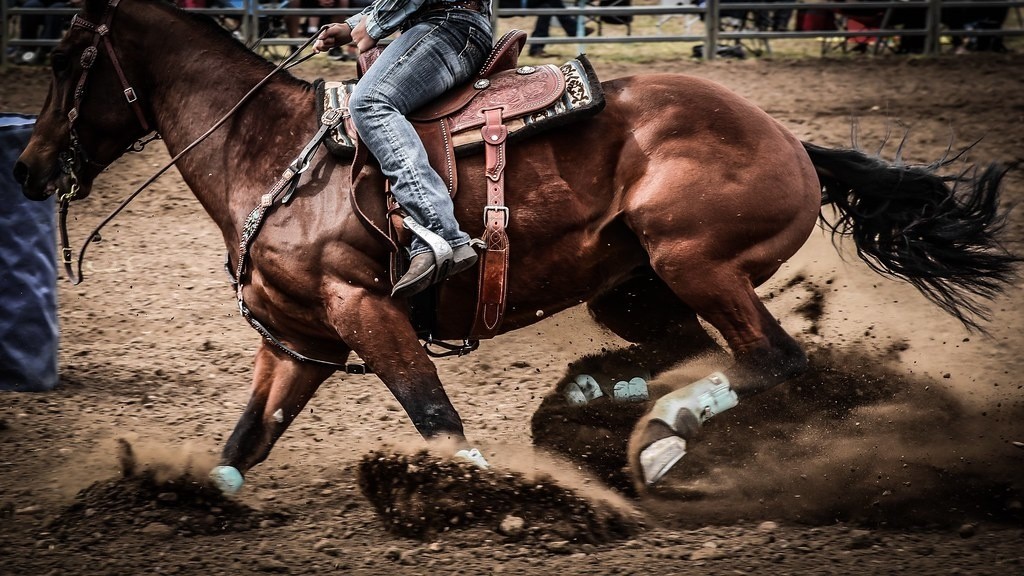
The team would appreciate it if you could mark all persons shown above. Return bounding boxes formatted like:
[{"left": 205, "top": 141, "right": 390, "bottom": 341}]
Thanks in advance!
[
  {"left": 184, "top": 0, "right": 1011, "bottom": 64},
  {"left": 312, "top": 0, "right": 494, "bottom": 300}
]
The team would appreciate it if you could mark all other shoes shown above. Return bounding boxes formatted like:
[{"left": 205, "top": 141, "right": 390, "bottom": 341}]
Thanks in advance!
[{"left": 392, "top": 242, "right": 475, "bottom": 296}]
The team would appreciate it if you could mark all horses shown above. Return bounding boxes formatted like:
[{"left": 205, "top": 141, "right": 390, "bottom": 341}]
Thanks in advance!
[{"left": 14, "top": 0, "right": 1023, "bottom": 498}]
